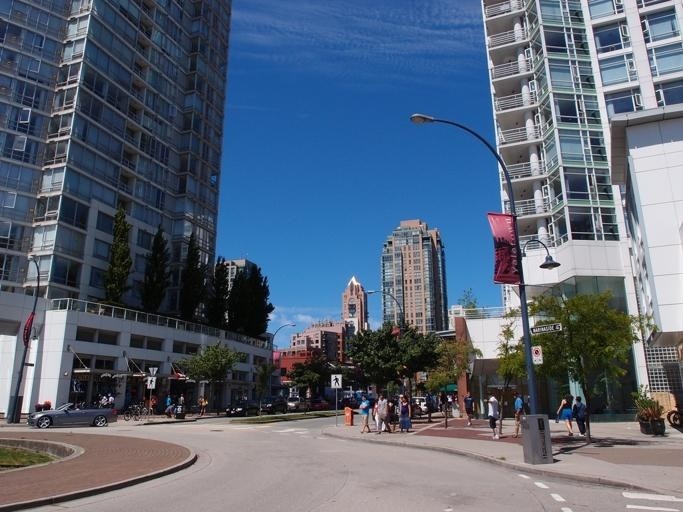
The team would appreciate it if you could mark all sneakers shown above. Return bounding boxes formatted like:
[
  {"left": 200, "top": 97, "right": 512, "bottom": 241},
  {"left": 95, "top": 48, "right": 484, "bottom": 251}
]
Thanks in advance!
[
  {"left": 359, "top": 430, "right": 372, "bottom": 433},
  {"left": 511, "top": 432, "right": 517, "bottom": 437},
  {"left": 376, "top": 431, "right": 381, "bottom": 434},
  {"left": 492, "top": 434, "right": 499, "bottom": 440},
  {"left": 577, "top": 432, "right": 585, "bottom": 436},
  {"left": 567, "top": 431, "right": 573, "bottom": 436}
]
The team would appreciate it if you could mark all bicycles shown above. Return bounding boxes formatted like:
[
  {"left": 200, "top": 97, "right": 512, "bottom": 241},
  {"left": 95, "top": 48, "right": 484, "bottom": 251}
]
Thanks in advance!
[{"left": 121, "top": 404, "right": 141, "bottom": 422}]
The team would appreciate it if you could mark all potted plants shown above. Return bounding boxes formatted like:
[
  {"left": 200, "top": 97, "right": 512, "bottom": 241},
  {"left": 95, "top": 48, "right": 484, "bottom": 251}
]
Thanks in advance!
[{"left": 628, "top": 382, "right": 667, "bottom": 437}]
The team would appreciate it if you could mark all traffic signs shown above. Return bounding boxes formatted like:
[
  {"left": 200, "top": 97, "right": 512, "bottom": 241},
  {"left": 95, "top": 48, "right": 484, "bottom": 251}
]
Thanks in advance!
[{"left": 529, "top": 323, "right": 562, "bottom": 336}]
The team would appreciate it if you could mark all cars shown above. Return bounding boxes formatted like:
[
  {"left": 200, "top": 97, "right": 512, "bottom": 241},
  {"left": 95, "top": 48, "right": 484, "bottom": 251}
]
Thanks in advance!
[{"left": 221, "top": 387, "right": 437, "bottom": 417}]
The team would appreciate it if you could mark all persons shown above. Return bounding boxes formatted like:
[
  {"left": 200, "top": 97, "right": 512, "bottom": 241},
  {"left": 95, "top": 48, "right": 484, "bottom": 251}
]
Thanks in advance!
[
  {"left": 73, "top": 392, "right": 208, "bottom": 419},
  {"left": 511, "top": 391, "right": 524, "bottom": 438},
  {"left": 463, "top": 391, "right": 474, "bottom": 426},
  {"left": 573, "top": 396, "right": 586, "bottom": 436},
  {"left": 357, "top": 390, "right": 457, "bottom": 433},
  {"left": 556, "top": 393, "right": 574, "bottom": 436},
  {"left": 483, "top": 390, "right": 499, "bottom": 440}
]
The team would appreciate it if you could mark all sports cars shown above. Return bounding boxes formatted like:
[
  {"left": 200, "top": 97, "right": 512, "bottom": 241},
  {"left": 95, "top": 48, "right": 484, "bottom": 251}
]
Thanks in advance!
[{"left": 27, "top": 402, "right": 118, "bottom": 429}]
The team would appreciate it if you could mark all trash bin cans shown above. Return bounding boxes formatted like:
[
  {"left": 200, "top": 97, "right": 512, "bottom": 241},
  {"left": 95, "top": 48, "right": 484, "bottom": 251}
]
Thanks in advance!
[
  {"left": 344, "top": 407, "right": 353, "bottom": 426},
  {"left": 520, "top": 416, "right": 553, "bottom": 464},
  {"left": 175, "top": 403, "right": 186, "bottom": 419},
  {"left": 7, "top": 396, "right": 23, "bottom": 423}
]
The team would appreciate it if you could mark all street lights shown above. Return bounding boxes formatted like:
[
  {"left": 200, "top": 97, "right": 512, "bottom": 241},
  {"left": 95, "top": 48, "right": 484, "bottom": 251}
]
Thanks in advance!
[
  {"left": 408, "top": 110, "right": 562, "bottom": 467},
  {"left": 6, "top": 252, "right": 41, "bottom": 424},
  {"left": 269, "top": 323, "right": 297, "bottom": 400},
  {"left": 361, "top": 287, "right": 413, "bottom": 420}
]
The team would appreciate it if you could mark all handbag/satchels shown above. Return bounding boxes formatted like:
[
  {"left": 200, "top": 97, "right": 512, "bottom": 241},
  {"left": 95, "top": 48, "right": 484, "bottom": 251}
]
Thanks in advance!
[{"left": 555, "top": 412, "right": 561, "bottom": 424}]
